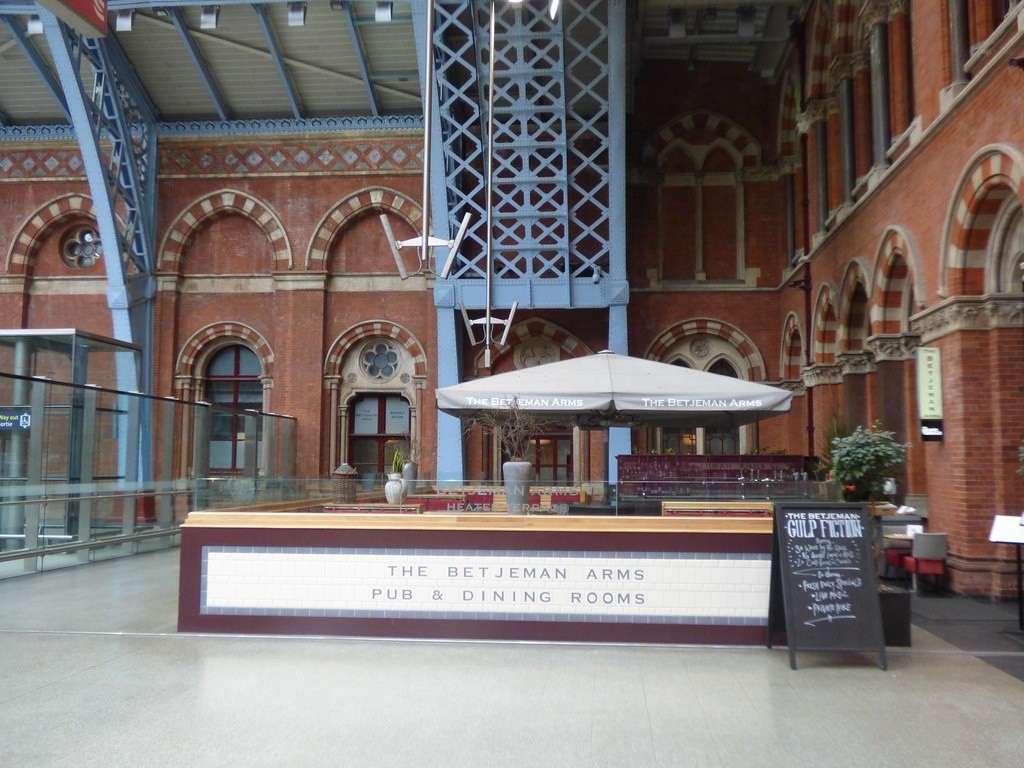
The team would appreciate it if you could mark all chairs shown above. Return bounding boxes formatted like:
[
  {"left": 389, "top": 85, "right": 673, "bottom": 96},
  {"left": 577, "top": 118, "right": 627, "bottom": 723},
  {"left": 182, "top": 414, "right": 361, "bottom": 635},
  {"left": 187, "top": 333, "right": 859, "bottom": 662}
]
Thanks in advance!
[
  {"left": 883, "top": 525, "right": 925, "bottom": 582},
  {"left": 903, "top": 532, "right": 948, "bottom": 594}
]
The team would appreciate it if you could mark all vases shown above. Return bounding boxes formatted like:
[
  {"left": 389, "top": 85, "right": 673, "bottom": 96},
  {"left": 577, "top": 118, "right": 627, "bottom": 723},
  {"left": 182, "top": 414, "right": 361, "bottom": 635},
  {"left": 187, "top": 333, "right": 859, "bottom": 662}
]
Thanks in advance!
[
  {"left": 503, "top": 461, "right": 532, "bottom": 515},
  {"left": 403, "top": 462, "right": 417, "bottom": 495},
  {"left": 842, "top": 479, "right": 871, "bottom": 501}
]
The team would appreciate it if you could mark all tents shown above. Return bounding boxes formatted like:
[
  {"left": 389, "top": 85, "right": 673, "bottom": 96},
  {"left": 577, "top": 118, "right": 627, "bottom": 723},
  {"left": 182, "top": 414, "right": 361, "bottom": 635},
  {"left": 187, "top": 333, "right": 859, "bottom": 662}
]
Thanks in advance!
[{"left": 435, "top": 350, "right": 793, "bottom": 430}]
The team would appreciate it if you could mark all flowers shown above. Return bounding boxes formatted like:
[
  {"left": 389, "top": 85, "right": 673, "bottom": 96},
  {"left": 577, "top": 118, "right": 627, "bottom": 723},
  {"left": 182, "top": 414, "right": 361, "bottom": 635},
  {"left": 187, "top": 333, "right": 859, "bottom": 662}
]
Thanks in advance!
[{"left": 823, "top": 418, "right": 914, "bottom": 492}]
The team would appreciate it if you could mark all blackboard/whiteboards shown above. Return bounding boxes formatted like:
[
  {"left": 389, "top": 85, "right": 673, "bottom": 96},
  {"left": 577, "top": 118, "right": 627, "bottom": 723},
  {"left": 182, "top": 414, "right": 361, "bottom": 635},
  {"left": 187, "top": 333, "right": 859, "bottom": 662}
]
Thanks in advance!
[{"left": 773, "top": 500, "right": 887, "bottom": 654}]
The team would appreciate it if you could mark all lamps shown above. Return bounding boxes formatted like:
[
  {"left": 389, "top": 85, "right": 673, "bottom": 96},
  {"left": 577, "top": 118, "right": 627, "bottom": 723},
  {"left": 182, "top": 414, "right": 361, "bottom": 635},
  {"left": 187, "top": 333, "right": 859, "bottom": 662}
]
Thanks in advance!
[
  {"left": 200, "top": 5, "right": 220, "bottom": 29},
  {"left": 287, "top": 5, "right": 307, "bottom": 27},
  {"left": 374, "top": 1, "right": 393, "bottom": 23},
  {"left": 115, "top": 8, "right": 137, "bottom": 32},
  {"left": 27, "top": 15, "right": 44, "bottom": 34}
]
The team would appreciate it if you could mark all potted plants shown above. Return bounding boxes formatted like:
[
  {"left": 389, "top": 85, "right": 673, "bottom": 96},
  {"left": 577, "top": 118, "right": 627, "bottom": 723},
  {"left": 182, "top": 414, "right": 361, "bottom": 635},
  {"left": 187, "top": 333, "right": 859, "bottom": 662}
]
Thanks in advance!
[{"left": 384, "top": 450, "right": 408, "bottom": 504}]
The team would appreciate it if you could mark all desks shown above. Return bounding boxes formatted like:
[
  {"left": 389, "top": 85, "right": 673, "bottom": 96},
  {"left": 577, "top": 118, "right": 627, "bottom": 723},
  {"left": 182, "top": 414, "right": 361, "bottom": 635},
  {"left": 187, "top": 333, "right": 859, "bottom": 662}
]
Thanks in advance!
[
  {"left": 882, "top": 534, "right": 913, "bottom": 591},
  {"left": 568, "top": 503, "right": 635, "bottom": 515}
]
